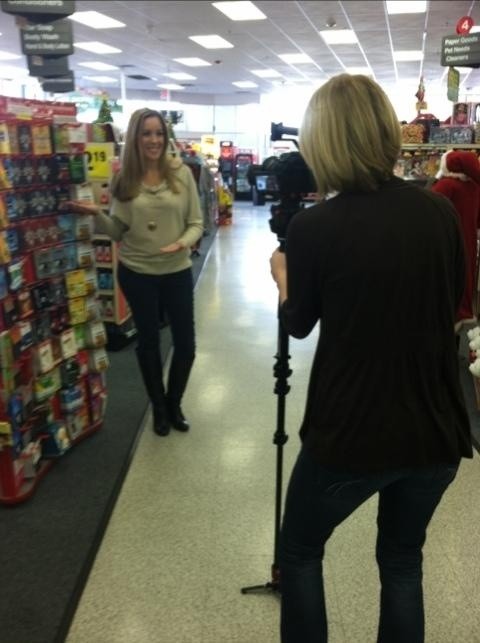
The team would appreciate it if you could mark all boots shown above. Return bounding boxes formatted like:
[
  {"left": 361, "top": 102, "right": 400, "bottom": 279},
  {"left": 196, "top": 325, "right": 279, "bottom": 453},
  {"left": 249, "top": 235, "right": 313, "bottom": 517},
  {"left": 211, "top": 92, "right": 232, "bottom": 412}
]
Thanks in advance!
[
  {"left": 132, "top": 346, "right": 172, "bottom": 436},
  {"left": 165, "top": 350, "right": 198, "bottom": 432}
]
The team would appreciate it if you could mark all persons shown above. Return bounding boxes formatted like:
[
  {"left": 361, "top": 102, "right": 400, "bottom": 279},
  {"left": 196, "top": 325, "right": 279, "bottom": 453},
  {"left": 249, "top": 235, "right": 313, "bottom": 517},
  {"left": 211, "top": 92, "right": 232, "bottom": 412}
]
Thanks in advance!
[
  {"left": 60, "top": 108, "right": 204, "bottom": 437},
  {"left": 268, "top": 72, "right": 478, "bottom": 641}
]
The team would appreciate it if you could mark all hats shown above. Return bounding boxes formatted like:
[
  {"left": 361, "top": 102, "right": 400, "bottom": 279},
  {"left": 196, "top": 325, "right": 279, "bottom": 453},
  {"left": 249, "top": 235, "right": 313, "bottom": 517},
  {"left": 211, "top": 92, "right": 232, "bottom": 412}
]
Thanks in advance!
[{"left": 441, "top": 147, "right": 480, "bottom": 182}]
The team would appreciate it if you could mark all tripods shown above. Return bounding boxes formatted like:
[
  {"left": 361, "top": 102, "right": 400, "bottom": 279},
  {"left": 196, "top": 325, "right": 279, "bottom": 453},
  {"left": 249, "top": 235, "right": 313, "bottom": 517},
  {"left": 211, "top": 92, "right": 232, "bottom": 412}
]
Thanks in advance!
[{"left": 240, "top": 204, "right": 307, "bottom": 595}]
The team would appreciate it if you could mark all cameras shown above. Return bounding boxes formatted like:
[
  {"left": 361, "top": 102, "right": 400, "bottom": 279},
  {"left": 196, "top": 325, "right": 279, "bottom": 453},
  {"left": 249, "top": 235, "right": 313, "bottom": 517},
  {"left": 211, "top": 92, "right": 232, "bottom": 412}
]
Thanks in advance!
[{"left": 246, "top": 149, "right": 329, "bottom": 203}]
